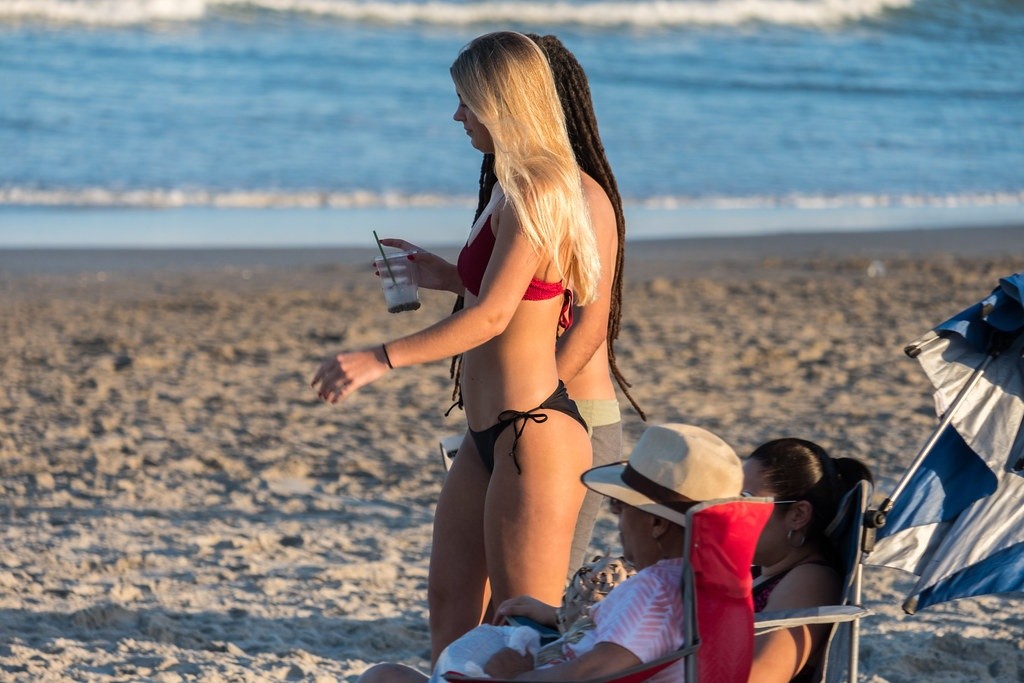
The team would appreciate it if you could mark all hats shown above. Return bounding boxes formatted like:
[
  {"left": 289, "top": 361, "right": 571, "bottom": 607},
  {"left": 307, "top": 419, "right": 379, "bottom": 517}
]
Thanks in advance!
[{"left": 580, "top": 418, "right": 744, "bottom": 528}]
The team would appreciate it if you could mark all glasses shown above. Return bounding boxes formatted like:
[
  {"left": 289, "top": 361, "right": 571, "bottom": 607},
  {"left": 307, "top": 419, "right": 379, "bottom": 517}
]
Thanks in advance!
[{"left": 610, "top": 496, "right": 636, "bottom": 514}]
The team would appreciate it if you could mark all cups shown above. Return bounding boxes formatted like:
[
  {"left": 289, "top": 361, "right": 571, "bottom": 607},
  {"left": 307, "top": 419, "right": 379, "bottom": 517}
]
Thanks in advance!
[{"left": 375, "top": 250, "right": 421, "bottom": 314}]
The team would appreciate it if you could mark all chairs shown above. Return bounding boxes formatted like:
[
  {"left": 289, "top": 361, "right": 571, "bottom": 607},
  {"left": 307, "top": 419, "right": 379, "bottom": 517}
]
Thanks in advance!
[
  {"left": 441, "top": 496, "right": 774, "bottom": 683},
  {"left": 503, "top": 480, "right": 873, "bottom": 683}
]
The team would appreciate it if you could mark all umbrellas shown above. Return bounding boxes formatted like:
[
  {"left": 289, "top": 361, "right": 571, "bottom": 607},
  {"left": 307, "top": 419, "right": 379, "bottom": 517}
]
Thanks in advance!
[{"left": 863, "top": 272, "right": 1024, "bottom": 616}]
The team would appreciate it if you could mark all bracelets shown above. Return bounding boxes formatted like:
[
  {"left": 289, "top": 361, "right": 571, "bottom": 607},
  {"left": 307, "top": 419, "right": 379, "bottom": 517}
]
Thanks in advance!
[{"left": 382, "top": 344, "right": 393, "bottom": 369}]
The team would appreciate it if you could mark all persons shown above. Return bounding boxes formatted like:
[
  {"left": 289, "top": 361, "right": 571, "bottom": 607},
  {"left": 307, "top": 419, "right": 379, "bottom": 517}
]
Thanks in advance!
[
  {"left": 360, "top": 423, "right": 744, "bottom": 682},
  {"left": 493, "top": 438, "right": 872, "bottom": 683},
  {"left": 312, "top": 31, "right": 593, "bottom": 673},
  {"left": 450, "top": 35, "right": 645, "bottom": 627}
]
